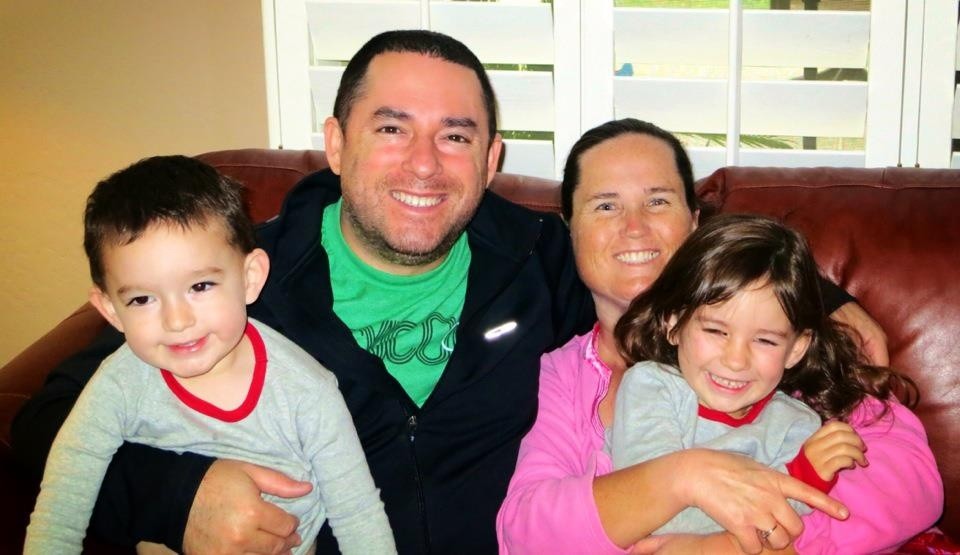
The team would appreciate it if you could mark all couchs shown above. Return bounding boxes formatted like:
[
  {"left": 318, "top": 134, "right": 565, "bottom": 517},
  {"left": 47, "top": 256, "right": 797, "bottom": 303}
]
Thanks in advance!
[{"left": 0, "top": 147, "right": 960, "bottom": 555}]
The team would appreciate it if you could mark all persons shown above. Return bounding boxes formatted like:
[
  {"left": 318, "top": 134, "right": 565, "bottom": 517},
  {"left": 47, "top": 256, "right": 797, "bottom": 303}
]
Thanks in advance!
[
  {"left": 612, "top": 215, "right": 922, "bottom": 539},
  {"left": 495, "top": 119, "right": 944, "bottom": 555},
  {"left": 22, "top": 154, "right": 400, "bottom": 555},
  {"left": 0, "top": 29, "right": 888, "bottom": 555}
]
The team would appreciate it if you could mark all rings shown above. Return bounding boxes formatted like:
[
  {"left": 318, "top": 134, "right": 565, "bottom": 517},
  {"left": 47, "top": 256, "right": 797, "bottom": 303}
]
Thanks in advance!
[{"left": 761, "top": 524, "right": 778, "bottom": 538}]
